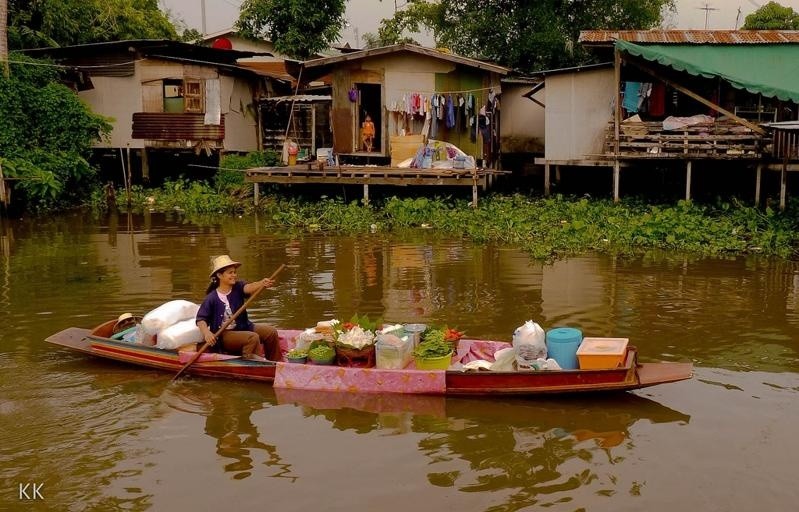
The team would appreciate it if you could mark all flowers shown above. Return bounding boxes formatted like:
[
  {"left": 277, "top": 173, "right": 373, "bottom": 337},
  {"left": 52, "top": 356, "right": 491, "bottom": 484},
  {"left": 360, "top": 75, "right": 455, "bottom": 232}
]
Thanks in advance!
[{"left": 442, "top": 323, "right": 467, "bottom": 341}]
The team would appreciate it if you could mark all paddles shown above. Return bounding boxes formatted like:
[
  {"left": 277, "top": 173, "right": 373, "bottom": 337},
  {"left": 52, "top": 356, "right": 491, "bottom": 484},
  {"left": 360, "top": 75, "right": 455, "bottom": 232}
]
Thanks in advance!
[{"left": 171, "top": 262, "right": 287, "bottom": 382}]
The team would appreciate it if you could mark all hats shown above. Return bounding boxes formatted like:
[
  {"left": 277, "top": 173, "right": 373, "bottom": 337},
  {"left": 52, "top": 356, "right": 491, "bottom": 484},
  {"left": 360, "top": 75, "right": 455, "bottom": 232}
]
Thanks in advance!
[{"left": 207, "top": 254, "right": 243, "bottom": 279}]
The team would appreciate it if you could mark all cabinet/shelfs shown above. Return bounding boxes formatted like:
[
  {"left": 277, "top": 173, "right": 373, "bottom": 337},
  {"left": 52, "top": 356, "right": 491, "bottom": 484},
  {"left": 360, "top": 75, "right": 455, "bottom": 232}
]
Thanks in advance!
[{"left": 734, "top": 105, "right": 777, "bottom": 157}]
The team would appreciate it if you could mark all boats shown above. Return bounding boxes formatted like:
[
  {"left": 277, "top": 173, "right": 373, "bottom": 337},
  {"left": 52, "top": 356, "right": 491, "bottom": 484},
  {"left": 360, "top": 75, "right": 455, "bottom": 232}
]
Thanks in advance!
[{"left": 44, "top": 326, "right": 694, "bottom": 394}]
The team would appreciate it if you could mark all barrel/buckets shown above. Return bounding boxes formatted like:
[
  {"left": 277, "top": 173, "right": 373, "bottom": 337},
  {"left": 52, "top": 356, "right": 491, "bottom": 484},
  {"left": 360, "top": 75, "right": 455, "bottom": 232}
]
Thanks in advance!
[{"left": 546, "top": 328, "right": 582, "bottom": 369}]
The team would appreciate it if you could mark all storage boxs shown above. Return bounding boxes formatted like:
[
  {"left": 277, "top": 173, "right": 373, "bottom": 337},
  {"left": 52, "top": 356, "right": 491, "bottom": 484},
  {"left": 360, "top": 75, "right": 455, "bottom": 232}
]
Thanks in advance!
[
  {"left": 575, "top": 337, "right": 629, "bottom": 370},
  {"left": 374, "top": 331, "right": 415, "bottom": 373}
]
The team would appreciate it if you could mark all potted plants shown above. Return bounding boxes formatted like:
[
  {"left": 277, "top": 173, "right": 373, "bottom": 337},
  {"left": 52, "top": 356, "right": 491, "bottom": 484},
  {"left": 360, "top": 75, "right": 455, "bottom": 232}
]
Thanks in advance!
[
  {"left": 442, "top": 339, "right": 467, "bottom": 351},
  {"left": 308, "top": 344, "right": 336, "bottom": 366},
  {"left": 407, "top": 331, "right": 452, "bottom": 371},
  {"left": 283, "top": 346, "right": 306, "bottom": 366}
]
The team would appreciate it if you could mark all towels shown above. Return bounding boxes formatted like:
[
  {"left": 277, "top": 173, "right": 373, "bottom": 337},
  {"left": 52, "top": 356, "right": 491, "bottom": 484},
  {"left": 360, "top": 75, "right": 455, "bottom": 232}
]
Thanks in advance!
[{"left": 622, "top": 81, "right": 641, "bottom": 113}]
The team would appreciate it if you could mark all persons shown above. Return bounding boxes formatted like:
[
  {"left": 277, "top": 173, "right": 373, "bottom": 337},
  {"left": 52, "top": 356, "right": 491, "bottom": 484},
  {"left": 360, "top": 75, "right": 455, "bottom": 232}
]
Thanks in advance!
[
  {"left": 362, "top": 115, "right": 376, "bottom": 153},
  {"left": 196, "top": 254, "right": 285, "bottom": 362}
]
